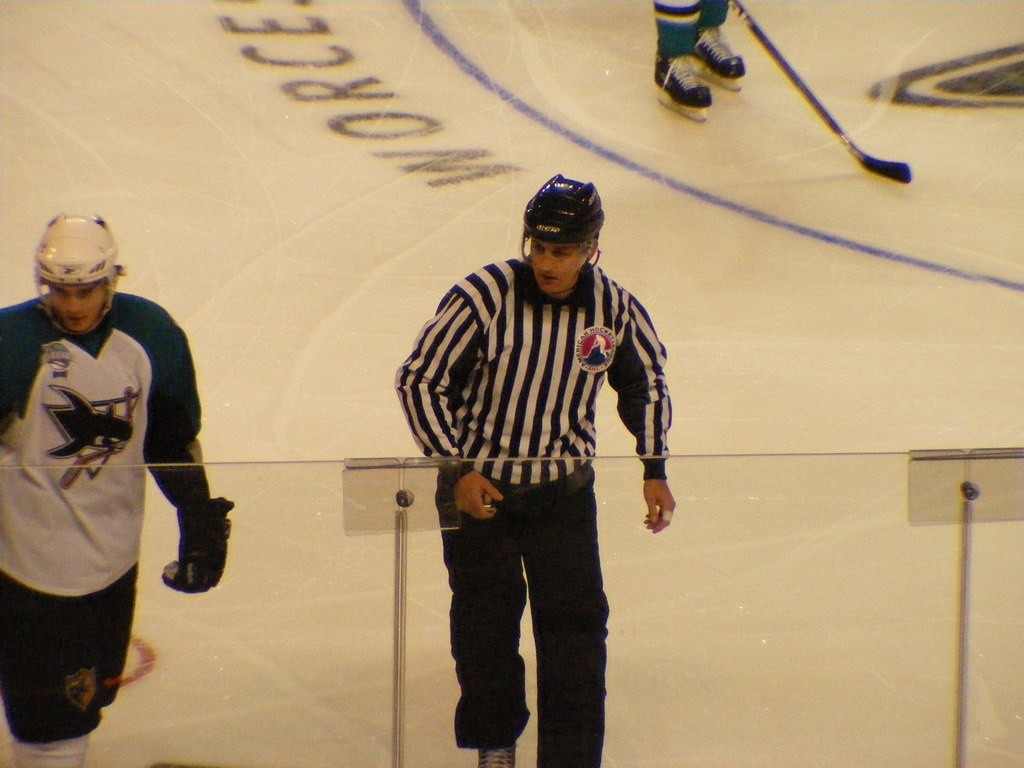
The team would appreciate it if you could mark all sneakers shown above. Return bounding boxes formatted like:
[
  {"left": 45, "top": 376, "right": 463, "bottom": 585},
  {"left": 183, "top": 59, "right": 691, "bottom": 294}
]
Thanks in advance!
[
  {"left": 654, "top": 51, "right": 711, "bottom": 121},
  {"left": 477, "top": 741, "right": 515, "bottom": 768},
  {"left": 692, "top": 27, "right": 745, "bottom": 92}
]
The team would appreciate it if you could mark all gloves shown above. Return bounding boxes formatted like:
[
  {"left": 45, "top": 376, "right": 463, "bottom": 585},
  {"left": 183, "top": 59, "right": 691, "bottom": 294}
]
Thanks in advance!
[{"left": 161, "top": 497, "right": 233, "bottom": 593}]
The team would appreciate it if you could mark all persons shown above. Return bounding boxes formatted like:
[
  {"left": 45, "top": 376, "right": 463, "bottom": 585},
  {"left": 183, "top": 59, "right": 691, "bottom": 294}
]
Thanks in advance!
[
  {"left": 0, "top": 213, "right": 234, "bottom": 768},
  {"left": 394, "top": 174, "right": 677, "bottom": 768},
  {"left": 654, "top": 0, "right": 747, "bottom": 122}
]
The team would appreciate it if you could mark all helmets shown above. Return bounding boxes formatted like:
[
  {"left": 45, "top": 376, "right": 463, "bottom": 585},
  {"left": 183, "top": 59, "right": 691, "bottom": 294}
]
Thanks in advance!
[
  {"left": 35, "top": 212, "right": 118, "bottom": 285},
  {"left": 522, "top": 172, "right": 604, "bottom": 246}
]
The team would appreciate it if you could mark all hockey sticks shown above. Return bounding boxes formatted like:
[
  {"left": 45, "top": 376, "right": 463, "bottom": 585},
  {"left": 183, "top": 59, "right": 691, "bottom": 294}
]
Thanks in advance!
[{"left": 730, "top": 0, "right": 915, "bottom": 185}]
